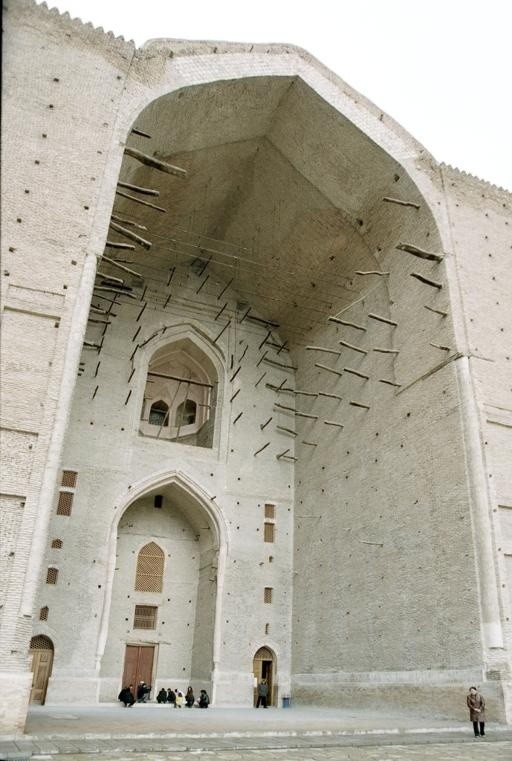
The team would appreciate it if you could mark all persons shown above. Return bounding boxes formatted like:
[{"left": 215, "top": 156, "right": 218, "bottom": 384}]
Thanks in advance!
[
  {"left": 256, "top": 679, "right": 269, "bottom": 708},
  {"left": 118, "top": 680, "right": 210, "bottom": 708},
  {"left": 467, "top": 686, "right": 486, "bottom": 738}
]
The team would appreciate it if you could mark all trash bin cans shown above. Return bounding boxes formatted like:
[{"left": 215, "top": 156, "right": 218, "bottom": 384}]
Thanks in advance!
[{"left": 283, "top": 698, "right": 290, "bottom": 709}]
